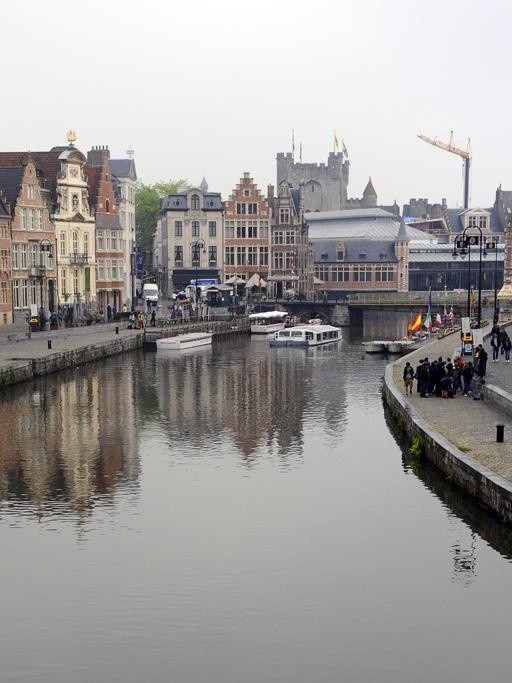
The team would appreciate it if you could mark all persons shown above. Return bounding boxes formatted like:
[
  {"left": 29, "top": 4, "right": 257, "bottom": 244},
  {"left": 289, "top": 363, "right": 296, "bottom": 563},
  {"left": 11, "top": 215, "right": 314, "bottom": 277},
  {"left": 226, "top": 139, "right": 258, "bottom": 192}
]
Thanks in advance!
[
  {"left": 491, "top": 324, "right": 512, "bottom": 362},
  {"left": 170, "top": 289, "right": 197, "bottom": 324},
  {"left": 23, "top": 297, "right": 157, "bottom": 329},
  {"left": 403, "top": 344, "right": 488, "bottom": 398},
  {"left": 493, "top": 311, "right": 498, "bottom": 327}
]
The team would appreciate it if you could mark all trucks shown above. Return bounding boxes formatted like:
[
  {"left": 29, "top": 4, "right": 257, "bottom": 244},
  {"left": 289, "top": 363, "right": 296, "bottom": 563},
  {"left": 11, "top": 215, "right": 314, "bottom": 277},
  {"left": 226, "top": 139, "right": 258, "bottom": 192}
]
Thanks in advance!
[{"left": 143, "top": 284, "right": 158, "bottom": 306}]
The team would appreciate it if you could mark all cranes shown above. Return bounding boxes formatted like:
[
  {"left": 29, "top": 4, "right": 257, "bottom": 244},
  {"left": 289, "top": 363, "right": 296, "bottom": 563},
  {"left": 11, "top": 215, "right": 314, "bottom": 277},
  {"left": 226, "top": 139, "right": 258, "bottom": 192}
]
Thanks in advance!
[{"left": 416, "top": 128, "right": 472, "bottom": 209}]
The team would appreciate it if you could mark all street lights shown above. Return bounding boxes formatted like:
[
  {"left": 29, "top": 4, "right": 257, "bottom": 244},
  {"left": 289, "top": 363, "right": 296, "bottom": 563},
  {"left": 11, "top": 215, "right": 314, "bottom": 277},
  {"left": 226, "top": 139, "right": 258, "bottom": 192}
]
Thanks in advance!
[
  {"left": 190, "top": 238, "right": 206, "bottom": 321},
  {"left": 452, "top": 225, "right": 497, "bottom": 329}
]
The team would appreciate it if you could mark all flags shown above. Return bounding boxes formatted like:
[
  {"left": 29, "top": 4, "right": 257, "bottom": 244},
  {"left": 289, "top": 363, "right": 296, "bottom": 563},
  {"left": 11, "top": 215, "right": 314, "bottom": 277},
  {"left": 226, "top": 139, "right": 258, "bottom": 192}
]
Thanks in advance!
[{"left": 404, "top": 311, "right": 442, "bottom": 334}]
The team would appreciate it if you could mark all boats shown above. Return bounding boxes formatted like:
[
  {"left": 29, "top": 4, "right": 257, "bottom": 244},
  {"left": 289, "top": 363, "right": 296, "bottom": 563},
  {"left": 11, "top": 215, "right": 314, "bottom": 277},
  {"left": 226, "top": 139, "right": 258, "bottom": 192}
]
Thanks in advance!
[
  {"left": 248, "top": 311, "right": 342, "bottom": 346},
  {"left": 156, "top": 332, "right": 212, "bottom": 350},
  {"left": 361, "top": 286, "right": 461, "bottom": 353}
]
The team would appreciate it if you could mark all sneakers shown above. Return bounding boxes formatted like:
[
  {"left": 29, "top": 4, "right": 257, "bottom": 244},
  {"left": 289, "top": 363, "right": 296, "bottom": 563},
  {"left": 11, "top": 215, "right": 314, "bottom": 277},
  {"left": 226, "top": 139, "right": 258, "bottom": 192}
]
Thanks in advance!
[
  {"left": 505, "top": 360, "right": 510, "bottom": 362},
  {"left": 492, "top": 359, "right": 499, "bottom": 362}
]
